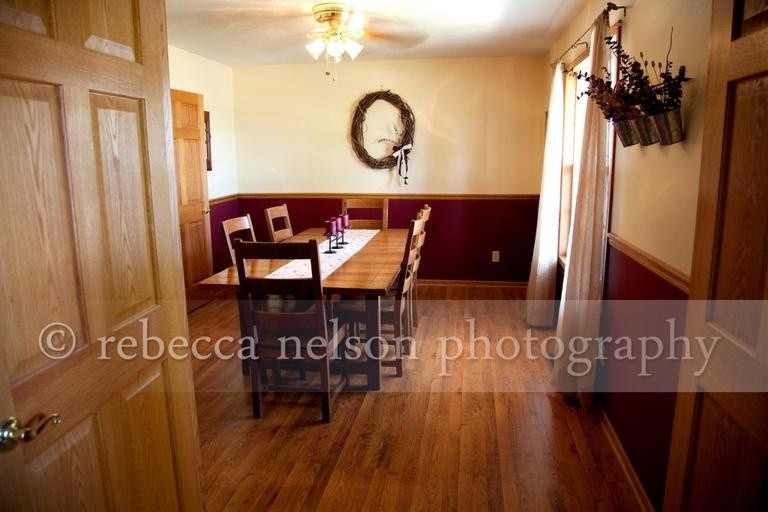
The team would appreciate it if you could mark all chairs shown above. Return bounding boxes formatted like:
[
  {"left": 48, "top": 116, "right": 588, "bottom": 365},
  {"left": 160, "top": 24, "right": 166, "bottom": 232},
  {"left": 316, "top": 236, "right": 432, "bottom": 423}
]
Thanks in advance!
[{"left": 199, "top": 198, "right": 432, "bottom": 425}]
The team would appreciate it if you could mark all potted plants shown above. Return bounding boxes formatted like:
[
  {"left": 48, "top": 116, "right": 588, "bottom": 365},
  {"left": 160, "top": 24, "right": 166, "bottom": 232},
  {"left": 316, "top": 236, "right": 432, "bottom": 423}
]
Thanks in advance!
[{"left": 561, "top": 27, "right": 686, "bottom": 145}]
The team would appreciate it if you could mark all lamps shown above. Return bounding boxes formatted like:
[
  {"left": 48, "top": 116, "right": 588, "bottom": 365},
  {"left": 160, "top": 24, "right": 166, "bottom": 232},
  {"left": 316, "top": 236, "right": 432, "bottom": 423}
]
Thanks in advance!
[{"left": 305, "top": 3, "right": 366, "bottom": 81}]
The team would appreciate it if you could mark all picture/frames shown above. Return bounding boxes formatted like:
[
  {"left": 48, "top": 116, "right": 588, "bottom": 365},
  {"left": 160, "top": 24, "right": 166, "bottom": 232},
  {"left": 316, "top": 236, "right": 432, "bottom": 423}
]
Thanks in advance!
[{"left": 204, "top": 111, "right": 212, "bottom": 170}]
